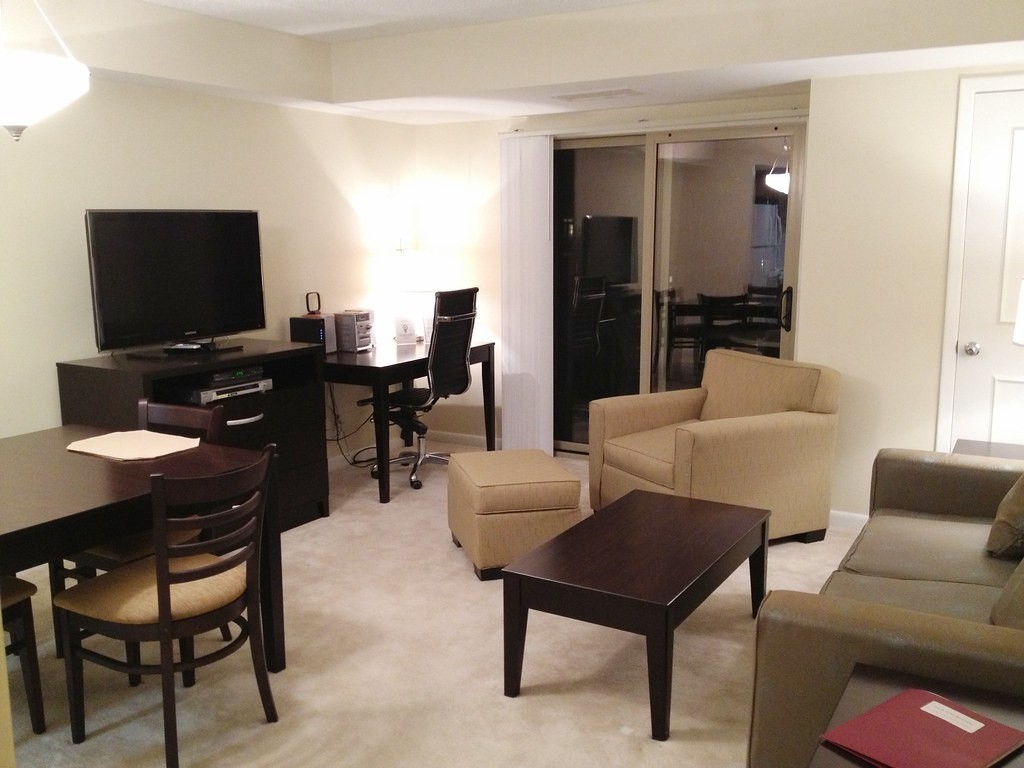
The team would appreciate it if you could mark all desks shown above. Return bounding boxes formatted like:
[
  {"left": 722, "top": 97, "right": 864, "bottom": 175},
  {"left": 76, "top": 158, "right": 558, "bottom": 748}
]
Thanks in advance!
[
  {"left": 0, "top": 424, "right": 287, "bottom": 674},
  {"left": 952, "top": 438, "right": 1024, "bottom": 462},
  {"left": 806, "top": 661, "right": 1024, "bottom": 768},
  {"left": 562, "top": 290, "right": 643, "bottom": 394},
  {"left": 318, "top": 340, "right": 497, "bottom": 503}
]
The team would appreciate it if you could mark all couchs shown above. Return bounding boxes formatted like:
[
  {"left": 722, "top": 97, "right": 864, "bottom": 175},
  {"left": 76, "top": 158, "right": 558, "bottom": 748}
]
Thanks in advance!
[
  {"left": 748, "top": 447, "right": 1024, "bottom": 768},
  {"left": 588, "top": 348, "right": 839, "bottom": 546}
]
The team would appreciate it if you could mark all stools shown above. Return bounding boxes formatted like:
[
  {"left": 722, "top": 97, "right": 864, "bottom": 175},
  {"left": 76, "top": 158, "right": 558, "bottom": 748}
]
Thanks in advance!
[{"left": 447, "top": 449, "right": 581, "bottom": 580}]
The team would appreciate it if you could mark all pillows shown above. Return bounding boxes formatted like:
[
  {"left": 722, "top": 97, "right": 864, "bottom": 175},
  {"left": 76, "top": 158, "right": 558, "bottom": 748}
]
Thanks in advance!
[
  {"left": 986, "top": 473, "right": 1024, "bottom": 557},
  {"left": 990, "top": 558, "right": 1024, "bottom": 630}
]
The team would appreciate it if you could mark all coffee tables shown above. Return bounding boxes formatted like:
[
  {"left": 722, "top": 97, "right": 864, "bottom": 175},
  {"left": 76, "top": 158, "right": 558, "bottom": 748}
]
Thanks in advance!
[{"left": 501, "top": 489, "right": 771, "bottom": 740}]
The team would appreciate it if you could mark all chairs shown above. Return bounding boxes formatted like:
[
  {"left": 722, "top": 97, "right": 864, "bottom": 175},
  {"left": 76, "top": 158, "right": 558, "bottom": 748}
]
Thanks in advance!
[
  {"left": 0, "top": 574, "right": 47, "bottom": 735},
  {"left": 358, "top": 287, "right": 479, "bottom": 490},
  {"left": 53, "top": 443, "right": 278, "bottom": 768},
  {"left": 566, "top": 274, "right": 607, "bottom": 392},
  {"left": 47, "top": 397, "right": 225, "bottom": 690},
  {"left": 664, "top": 285, "right": 782, "bottom": 383}
]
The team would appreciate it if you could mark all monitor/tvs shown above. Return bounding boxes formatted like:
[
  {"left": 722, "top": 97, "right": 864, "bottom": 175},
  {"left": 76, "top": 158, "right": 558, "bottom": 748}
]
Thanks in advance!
[
  {"left": 583, "top": 216, "right": 633, "bottom": 289},
  {"left": 84, "top": 208, "right": 266, "bottom": 355}
]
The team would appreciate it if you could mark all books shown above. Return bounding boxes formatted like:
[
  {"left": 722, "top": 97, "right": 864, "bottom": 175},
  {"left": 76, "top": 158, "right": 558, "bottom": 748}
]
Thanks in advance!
[{"left": 819, "top": 687, "right": 1024, "bottom": 768}]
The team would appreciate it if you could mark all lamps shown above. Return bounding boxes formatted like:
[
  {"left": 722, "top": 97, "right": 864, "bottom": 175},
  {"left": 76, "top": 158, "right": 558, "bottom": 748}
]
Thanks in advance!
[{"left": 766, "top": 140, "right": 791, "bottom": 194}]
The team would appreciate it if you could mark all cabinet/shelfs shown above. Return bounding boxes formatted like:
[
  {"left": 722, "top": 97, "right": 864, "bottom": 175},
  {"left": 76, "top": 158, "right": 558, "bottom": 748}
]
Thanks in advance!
[{"left": 56, "top": 337, "right": 331, "bottom": 533}]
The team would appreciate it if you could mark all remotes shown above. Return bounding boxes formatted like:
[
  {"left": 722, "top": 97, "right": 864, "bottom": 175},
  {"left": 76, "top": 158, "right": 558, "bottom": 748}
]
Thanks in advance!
[
  {"left": 162, "top": 343, "right": 202, "bottom": 353},
  {"left": 126, "top": 352, "right": 169, "bottom": 360}
]
